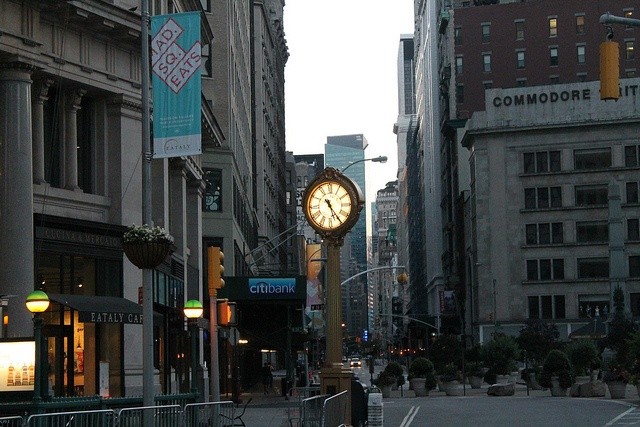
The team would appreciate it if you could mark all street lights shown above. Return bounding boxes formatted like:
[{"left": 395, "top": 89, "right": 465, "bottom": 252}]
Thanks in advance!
[
  {"left": 378, "top": 314, "right": 441, "bottom": 336},
  {"left": 26, "top": 291, "right": 50, "bottom": 427},
  {"left": 322, "top": 156, "right": 388, "bottom": 308},
  {"left": 183, "top": 299, "right": 203, "bottom": 426},
  {"left": 475, "top": 263, "right": 491, "bottom": 324},
  {"left": 325, "top": 266, "right": 405, "bottom": 304}
]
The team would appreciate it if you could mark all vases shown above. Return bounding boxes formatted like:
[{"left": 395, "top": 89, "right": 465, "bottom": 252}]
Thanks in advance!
[{"left": 121, "top": 241, "right": 174, "bottom": 269}]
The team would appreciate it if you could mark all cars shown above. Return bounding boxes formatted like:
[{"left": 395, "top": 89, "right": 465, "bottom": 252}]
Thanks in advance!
[{"left": 350, "top": 358, "right": 361, "bottom": 367}]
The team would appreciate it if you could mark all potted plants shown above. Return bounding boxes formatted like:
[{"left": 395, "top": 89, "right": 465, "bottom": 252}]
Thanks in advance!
[
  {"left": 544, "top": 349, "right": 575, "bottom": 397},
  {"left": 632, "top": 376, "right": 640, "bottom": 399},
  {"left": 603, "top": 359, "right": 630, "bottom": 398},
  {"left": 440, "top": 362, "right": 465, "bottom": 396},
  {"left": 372, "top": 376, "right": 397, "bottom": 392},
  {"left": 519, "top": 320, "right": 561, "bottom": 389},
  {"left": 407, "top": 357, "right": 436, "bottom": 397},
  {"left": 467, "top": 362, "right": 485, "bottom": 389},
  {"left": 478, "top": 334, "right": 522, "bottom": 386}
]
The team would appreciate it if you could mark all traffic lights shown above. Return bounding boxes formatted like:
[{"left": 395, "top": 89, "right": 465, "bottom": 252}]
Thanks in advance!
[
  {"left": 208, "top": 247, "right": 226, "bottom": 288},
  {"left": 218, "top": 303, "right": 231, "bottom": 326}
]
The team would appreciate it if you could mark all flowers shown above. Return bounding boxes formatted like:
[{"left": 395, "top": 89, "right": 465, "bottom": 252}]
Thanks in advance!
[{"left": 121, "top": 221, "right": 174, "bottom": 242}]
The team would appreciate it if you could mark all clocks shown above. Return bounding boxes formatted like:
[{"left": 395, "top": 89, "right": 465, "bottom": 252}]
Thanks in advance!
[{"left": 301, "top": 166, "right": 364, "bottom": 238}]
